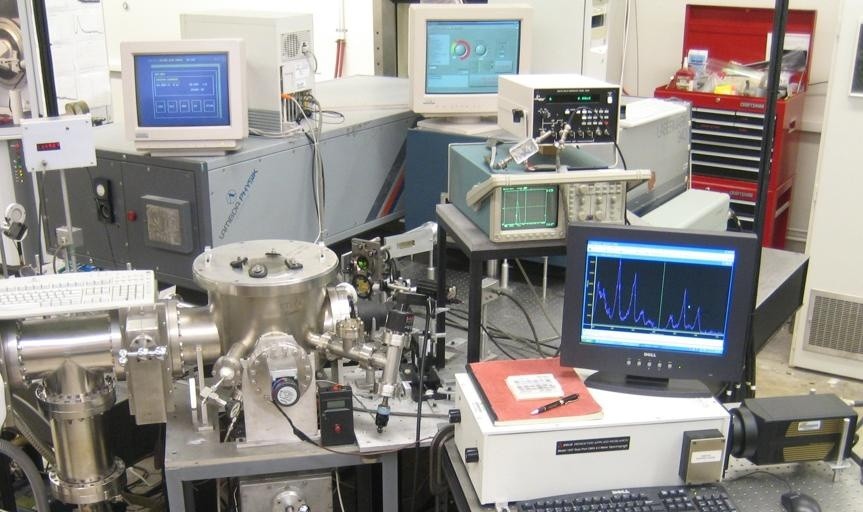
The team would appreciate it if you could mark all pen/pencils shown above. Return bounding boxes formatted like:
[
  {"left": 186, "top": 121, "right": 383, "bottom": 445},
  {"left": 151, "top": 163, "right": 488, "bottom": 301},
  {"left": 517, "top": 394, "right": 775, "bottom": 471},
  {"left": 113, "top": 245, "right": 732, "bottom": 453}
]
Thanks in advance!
[{"left": 530, "top": 393, "right": 580, "bottom": 415}]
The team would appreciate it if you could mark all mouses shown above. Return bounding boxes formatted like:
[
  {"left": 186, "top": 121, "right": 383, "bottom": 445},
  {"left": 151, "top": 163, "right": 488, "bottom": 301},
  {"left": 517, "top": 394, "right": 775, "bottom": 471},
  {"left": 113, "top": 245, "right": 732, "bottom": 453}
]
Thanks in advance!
[{"left": 781, "top": 490, "right": 822, "bottom": 512}]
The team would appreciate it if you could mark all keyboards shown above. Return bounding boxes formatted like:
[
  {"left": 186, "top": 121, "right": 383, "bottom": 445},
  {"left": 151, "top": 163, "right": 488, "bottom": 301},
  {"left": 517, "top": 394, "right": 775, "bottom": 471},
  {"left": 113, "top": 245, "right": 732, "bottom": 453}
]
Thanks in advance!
[
  {"left": 516, "top": 482, "right": 741, "bottom": 512},
  {"left": 0, "top": 270, "right": 154, "bottom": 320}
]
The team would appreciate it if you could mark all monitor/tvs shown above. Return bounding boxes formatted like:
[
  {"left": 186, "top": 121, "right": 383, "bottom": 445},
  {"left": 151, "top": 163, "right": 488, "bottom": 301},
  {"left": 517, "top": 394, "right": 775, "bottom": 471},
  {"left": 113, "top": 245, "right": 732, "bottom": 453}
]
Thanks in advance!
[
  {"left": 560, "top": 222, "right": 759, "bottom": 398},
  {"left": 120, "top": 38, "right": 247, "bottom": 159},
  {"left": 408, "top": 4, "right": 536, "bottom": 135}
]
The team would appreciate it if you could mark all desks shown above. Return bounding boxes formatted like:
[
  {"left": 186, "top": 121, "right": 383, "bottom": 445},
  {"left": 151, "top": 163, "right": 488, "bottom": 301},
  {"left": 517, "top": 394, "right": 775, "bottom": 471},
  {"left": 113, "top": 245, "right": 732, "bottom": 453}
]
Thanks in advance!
[
  {"left": 439, "top": 414, "right": 863, "bottom": 512},
  {"left": 164, "top": 352, "right": 399, "bottom": 512},
  {"left": 434, "top": 203, "right": 658, "bottom": 371}
]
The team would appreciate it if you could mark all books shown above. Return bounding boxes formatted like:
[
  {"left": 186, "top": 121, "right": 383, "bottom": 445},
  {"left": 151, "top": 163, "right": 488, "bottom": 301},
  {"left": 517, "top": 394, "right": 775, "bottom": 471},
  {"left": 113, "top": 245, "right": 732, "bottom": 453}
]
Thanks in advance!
[{"left": 465, "top": 356, "right": 604, "bottom": 426}]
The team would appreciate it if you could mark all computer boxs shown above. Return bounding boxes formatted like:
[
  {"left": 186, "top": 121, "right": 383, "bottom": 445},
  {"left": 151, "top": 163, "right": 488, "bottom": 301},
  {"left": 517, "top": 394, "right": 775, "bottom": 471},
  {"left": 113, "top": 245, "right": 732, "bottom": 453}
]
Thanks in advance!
[{"left": 179, "top": 14, "right": 318, "bottom": 137}]
